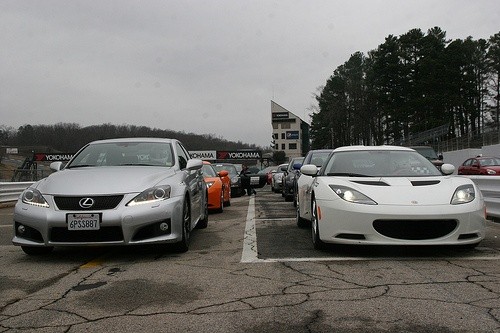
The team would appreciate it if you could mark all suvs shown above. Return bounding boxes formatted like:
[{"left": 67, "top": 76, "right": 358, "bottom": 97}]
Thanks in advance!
[{"left": 405, "top": 146, "right": 444, "bottom": 175}]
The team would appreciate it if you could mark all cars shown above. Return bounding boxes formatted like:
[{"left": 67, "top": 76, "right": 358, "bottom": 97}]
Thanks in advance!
[
  {"left": 199, "top": 162, "right": 267, "bottom": 212},
  {"left": 458, "top": 157, "right": 500, "bottom": 176},
  {"left": 11, "top": 138, "right": 209, "bottom": 255},
  {"left": 300, "top": 145, "right": 486, "bottom": 252},
  {"left": 267, "top": 150, "right": 369, "bottom": 202}
]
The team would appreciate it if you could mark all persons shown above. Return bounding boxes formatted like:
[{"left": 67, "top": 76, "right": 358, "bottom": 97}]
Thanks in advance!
[{"left": 238, "top": 164, "right": 256, "bottom": 196}]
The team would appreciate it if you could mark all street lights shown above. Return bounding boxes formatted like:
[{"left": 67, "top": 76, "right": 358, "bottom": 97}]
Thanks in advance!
[{"left": 322, "top": 127, "right": 334, "bottom": 149}]
